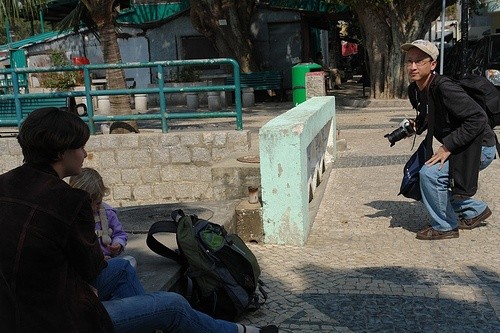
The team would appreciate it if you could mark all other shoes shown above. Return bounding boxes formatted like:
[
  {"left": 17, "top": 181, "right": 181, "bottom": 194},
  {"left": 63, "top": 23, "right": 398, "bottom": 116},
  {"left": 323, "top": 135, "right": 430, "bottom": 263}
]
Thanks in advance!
[{"left": 241, "top": 324, "right": 278, "bottom": 333}]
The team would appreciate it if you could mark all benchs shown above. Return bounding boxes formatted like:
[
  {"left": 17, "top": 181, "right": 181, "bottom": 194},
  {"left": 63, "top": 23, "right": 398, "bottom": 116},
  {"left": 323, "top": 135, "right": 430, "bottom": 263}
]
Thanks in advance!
[{"left": 215, "top": 69, "right": 284, "bottom": 103}]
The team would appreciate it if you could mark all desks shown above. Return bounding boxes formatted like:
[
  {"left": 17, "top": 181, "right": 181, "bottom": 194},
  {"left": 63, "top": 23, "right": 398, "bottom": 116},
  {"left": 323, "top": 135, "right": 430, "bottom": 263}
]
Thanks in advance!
[
  {"left": 92, "top": 78, "right": 135, "bottom": 90},
  {"left": 199, "top": 72, "right": 233, "bottom": 107}
]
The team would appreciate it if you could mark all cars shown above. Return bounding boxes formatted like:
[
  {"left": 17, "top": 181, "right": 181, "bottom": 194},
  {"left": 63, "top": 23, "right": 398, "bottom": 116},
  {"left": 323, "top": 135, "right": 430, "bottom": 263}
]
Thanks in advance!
[{"left": 432, "top": 33, "right": 500, "bottom": 93}]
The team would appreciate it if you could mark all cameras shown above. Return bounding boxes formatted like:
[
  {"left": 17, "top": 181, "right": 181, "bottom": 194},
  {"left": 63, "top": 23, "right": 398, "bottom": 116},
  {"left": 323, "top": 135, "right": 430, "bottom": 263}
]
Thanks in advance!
[{"left": 384, "top": 122, "right": 415, "bottom": 147}]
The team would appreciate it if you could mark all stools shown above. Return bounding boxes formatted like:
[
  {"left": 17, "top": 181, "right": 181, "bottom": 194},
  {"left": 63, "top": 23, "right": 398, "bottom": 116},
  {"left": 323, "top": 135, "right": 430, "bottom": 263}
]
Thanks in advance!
[
  {"left": 134, "top": 93, "right": 148, "bottom": 112},
  {"left": 81, "top": 95, "right": 96, "bottom": 113},
  {"left": 183, "top": 91, "right": 199, "bottom": 110},
  {"left": 241, "top": 87, "right": 255, "bottom": 107},
  {"left": 207, "top": 92, "right": 221, "bottom": 111},
  {"left": 97, "top": 96, "right": 110, "bottom": 114}
]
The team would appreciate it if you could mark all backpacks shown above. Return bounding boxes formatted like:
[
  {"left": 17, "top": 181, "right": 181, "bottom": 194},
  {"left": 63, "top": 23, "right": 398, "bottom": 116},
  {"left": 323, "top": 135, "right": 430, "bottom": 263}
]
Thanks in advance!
[
  {"left": 147, "top": 209, "right": 270, "bottom": 322},
  {"left": 410, "top": 74, "right": 500, "bottom": 129}
]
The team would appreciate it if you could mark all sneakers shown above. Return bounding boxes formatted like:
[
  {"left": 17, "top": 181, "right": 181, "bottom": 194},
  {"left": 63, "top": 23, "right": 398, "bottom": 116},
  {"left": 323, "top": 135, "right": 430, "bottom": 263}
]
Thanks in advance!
[
  {"left": 417, "top": 227, "right": 459, "bottom": 239},
  {"left": 458, "top": 206, "right": 492, "bottom": 230}
]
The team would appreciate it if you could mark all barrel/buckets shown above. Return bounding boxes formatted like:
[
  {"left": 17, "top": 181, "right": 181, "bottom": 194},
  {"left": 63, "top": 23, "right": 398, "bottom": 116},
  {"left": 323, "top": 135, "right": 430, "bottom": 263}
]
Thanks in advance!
[{"left": 291, "top": 61, "right": 322, "bottom": 107}]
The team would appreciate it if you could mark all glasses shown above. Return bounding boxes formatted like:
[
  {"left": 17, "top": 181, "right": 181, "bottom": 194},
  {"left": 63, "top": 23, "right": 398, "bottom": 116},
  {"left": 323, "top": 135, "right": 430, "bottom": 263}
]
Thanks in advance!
[{"left": 404, "top": 58, "right": 432, "bottom": 66}]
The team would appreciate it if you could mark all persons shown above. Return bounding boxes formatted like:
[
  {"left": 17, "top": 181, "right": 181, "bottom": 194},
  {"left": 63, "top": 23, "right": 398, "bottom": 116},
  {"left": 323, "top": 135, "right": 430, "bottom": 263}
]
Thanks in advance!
[
  {"left": 69, "top": 167, "right": 128, "bottom": 260},
  {"left": 0, "top": 107, "right": 278, "bottom": 333},
  {"left": 400, "top": 39, "right": 496, "bottom": 240}
]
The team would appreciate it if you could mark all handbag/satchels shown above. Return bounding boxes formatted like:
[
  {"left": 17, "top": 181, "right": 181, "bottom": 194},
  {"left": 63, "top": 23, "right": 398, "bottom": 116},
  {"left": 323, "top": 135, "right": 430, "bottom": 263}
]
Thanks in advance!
[{"left": 397, "top": 139, "right": 434, "bottom": 203}]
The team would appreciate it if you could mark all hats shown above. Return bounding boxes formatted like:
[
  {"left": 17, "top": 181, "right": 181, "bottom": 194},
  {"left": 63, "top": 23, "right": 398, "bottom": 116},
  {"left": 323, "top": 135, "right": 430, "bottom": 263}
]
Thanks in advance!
[{"left": 400, "top": 39, "right": 439, "bottom": 61}]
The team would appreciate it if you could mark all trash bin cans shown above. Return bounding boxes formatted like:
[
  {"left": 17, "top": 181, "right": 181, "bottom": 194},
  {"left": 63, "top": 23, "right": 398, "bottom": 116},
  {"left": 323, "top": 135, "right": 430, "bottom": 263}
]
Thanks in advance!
[{"left": 292, "top": 63, "right": 321, "bottom": 107}]
